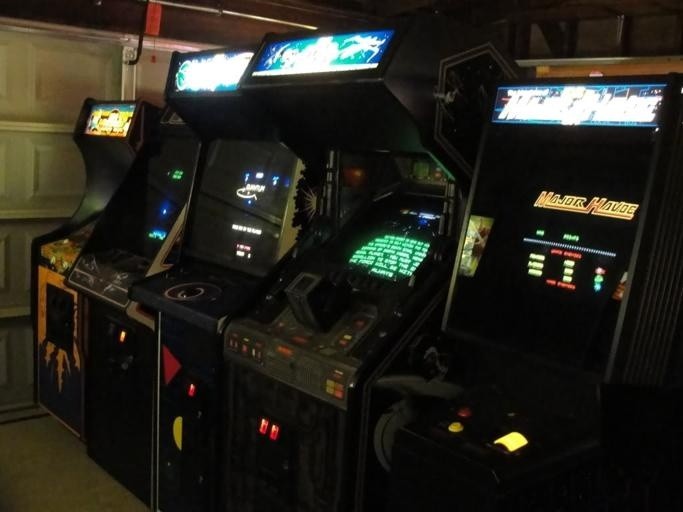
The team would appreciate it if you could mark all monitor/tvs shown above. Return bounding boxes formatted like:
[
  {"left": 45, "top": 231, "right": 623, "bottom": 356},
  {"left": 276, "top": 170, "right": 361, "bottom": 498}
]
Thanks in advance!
[
  {"left": 458, "top": 179, "right": 642, "bottom": 375},
  {"left": 305, "top": 191, "right": 446, "bottom": 288},
  {"left": 108, "top": 146, "right": 194, "bottom": 260},
  {"left": 185, "top": 137, "right": 306, "bottom": 279}
]
